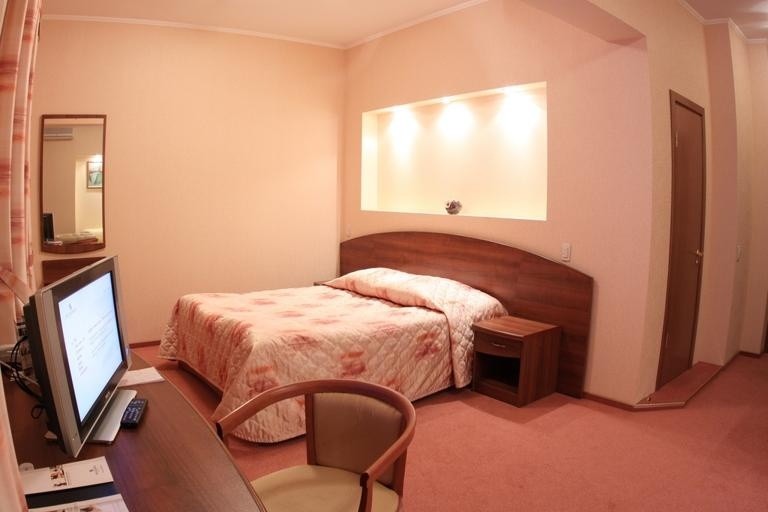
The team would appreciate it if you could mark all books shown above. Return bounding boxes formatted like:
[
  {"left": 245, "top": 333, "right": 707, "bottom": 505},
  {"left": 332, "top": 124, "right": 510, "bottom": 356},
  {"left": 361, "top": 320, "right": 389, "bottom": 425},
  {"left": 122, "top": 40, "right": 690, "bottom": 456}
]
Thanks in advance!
[{"left": 21, "top": 454, "right": 131, "bottom": 512}]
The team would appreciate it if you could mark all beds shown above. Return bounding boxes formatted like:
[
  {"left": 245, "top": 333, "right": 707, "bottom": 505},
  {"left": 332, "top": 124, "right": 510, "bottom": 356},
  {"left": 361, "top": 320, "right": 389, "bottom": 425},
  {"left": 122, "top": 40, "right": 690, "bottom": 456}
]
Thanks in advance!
[{"left": 156, "top": 230, "right": 594, "bottom": 443}]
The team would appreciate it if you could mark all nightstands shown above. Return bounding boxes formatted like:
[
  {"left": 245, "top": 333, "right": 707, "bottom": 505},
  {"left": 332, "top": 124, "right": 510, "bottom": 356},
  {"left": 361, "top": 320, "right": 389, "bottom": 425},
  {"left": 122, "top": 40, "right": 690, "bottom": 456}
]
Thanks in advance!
[{"left": 471, "top": 315, "right": 563, "bottom": 408}]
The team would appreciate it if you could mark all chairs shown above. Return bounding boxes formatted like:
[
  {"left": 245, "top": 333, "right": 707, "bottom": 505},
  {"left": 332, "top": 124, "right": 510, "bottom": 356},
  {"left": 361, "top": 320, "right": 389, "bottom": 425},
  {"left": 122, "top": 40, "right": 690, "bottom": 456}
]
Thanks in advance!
[{"left": 215, "top": 377, "right": 418, "bottom": 512}]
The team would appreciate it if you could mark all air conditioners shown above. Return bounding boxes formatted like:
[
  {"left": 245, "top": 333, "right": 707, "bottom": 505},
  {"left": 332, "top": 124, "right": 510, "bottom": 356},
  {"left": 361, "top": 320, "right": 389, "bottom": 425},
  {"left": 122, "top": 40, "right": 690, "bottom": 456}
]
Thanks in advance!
[{"left": 43, "top": 127, "right": 73, "bottom": 141}]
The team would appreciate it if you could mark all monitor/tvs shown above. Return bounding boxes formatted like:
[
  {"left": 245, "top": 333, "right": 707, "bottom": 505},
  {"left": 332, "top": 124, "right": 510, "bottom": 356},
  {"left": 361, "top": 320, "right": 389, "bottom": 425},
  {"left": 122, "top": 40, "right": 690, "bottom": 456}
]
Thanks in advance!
[{"left": 22, "top": 253, "right": 138, "bottom": 461}]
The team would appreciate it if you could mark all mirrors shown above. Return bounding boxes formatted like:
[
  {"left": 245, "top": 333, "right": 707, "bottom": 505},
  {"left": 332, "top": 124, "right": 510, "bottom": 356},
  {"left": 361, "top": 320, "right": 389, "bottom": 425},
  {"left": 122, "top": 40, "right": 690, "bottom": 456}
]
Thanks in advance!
[{"left": 40, "top": 113, "right": 106, "bottom": 254}]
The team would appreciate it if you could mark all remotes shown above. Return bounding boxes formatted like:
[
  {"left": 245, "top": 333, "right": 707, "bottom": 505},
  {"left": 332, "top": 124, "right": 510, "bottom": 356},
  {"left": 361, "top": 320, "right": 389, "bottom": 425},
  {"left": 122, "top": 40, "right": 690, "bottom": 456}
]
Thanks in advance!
[{"left": 121, "top": 398, "right": 149, "bottom": 427}]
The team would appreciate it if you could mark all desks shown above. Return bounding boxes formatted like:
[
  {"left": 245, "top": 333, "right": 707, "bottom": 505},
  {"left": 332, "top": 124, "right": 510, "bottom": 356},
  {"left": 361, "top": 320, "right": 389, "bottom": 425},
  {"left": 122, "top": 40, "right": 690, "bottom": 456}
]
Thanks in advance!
[{"left": 0, "top": 344, "right": 268, "bottom": 512}]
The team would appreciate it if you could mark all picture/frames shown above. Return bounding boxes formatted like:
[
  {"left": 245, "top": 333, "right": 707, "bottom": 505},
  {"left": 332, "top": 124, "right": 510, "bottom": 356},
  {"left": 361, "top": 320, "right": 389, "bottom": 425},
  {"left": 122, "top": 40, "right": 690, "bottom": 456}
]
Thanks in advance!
[{"left": 86, "top": 160, "right": 104, "bottom": 189}]
